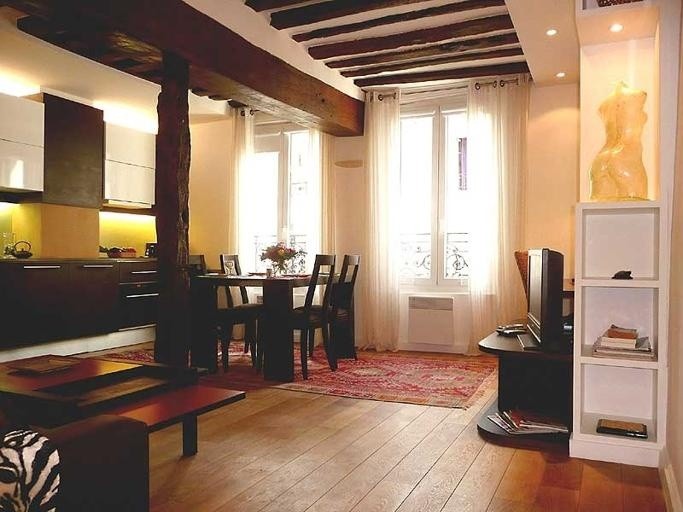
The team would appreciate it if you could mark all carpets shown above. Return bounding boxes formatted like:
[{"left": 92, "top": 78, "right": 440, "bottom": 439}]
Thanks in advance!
[{"left": 70, "top": 338, "right": 498, "bottom": 409}]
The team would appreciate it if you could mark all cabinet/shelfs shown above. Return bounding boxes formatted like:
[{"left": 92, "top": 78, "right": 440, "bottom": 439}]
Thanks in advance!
[
  {"left": 1, "top": 92, "right": 45, "bottom": 194},
  {"left": 573, "top": 201, "right": 667, "bottom": 451},
  {"left": 477, "top": 319, "right": 572, "bottom": 454},
  {"left": 118, "top": 262, "right": 159, "bottom": 331},
  {"left": 0, "top": 259, "right": 120, "bottom": 350},
  {"left": 104, "top": 122, "right": 155, "bottom": 210}
]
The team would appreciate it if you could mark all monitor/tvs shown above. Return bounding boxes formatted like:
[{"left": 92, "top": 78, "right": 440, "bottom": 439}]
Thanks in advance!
[{"left": 516, "top": 248, "right": 563, "bottom": 350}]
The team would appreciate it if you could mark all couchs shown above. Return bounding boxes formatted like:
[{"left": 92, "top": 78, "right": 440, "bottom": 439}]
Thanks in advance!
[{"left": 1, "top": 416, "right": 150, "bottom": 512}]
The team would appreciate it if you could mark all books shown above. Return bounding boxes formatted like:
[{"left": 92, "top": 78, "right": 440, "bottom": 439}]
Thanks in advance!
[
  {"left": 596, "top": 419, "right": 648, "bottom": 438},
  {"left": 593, "top": 324, "right": 655, "bottom": 360},
  {"left": 487, "top": 408, "right": 568, "bottom": 435}
]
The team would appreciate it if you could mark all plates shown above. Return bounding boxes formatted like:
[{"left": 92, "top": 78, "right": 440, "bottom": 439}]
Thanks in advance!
[{"left": 205, "top": 272, "right": 340, "bottom": 276}]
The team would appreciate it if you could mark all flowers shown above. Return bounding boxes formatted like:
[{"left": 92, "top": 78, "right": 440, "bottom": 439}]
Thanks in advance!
[{"left": 261, "top": 242, "right": 297, "bottom": 264}]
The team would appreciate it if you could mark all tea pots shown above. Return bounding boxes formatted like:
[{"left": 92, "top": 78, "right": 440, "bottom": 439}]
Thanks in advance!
[{"left": 12, "top": 242, "right": 32, "bottom": 258}]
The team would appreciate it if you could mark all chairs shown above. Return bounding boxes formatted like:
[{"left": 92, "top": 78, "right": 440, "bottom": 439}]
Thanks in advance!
[{"left": 189, "top": 254, "right": 360, "bottom": 380}]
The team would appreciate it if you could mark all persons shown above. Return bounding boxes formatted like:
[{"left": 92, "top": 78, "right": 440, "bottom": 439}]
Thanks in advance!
[{"left": 588, "top": 78, "right": 650, "bottom": 203}]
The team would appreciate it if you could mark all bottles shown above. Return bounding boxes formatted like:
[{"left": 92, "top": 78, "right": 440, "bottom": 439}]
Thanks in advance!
[{"left": 267, "top": 269, "right": 272, "bottom": 277}]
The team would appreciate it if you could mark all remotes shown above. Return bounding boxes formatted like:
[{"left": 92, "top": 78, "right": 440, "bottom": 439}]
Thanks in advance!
[
  {"left": 503, "top": 330, "right": 525, "bottom": 336},
  {"left": 496, "top": 328, "right": 527, "bottom": 334},
  {"left": 498, "top": 323, "right": 523, "bottom": 329}
]
